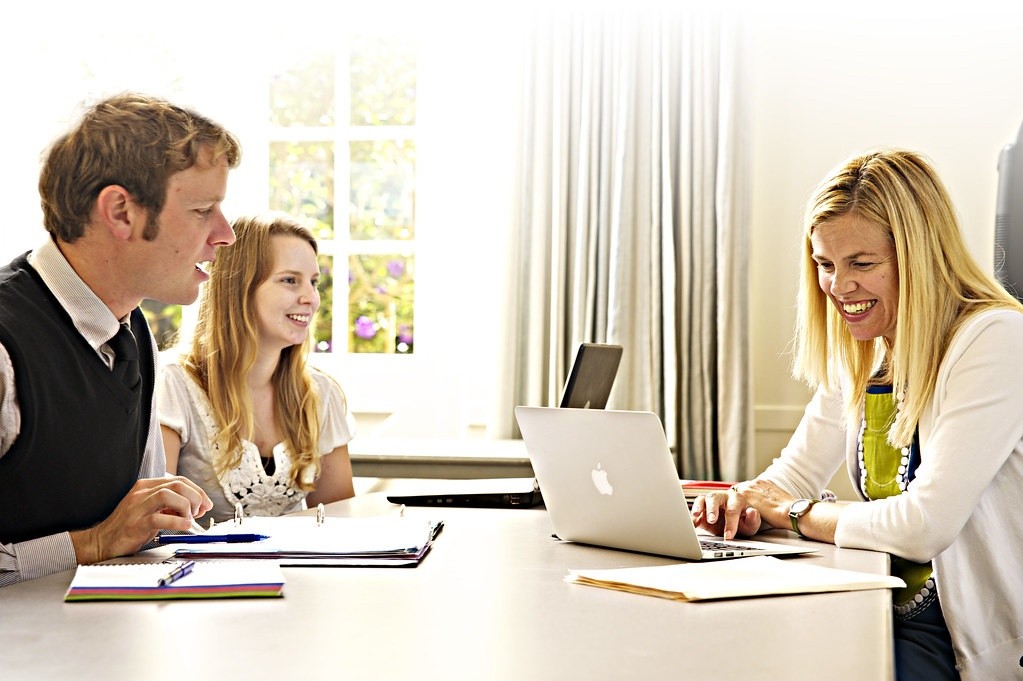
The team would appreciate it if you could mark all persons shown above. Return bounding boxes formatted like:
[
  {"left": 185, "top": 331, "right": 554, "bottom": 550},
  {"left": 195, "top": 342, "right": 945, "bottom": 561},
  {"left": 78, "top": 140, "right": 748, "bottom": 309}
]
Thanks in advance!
[
  {"left": 691, "top": 150, "right": 1023, "bottom": 681},
  {"left": 0, "top": 89, "right": 237, "bottom": 588},
  {"left": 155, "top": 211, "right": 357, "bottom": 528}
]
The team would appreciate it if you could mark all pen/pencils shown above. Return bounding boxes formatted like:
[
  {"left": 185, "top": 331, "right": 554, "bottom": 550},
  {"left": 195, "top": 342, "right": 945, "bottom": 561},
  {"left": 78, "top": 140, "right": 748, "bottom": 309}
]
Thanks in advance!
[
  {"left": 151, "top": 534, "right": 271, "bottom": 545},
  {"left": 157, "top": 561, "right": 194, "bottom": 586}
]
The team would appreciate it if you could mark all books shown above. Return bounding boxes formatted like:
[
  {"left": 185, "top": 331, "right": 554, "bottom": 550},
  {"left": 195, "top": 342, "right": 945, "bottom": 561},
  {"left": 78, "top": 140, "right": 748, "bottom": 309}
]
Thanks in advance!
[
  {"left": 64, "top": 560, "right": 286, "bottom": 602},
  {"left": 681, "top": 480, "right": 738, "bottom": 510},
  {"left": 167, "top": 503, "right": 445, "bottom": 568}
]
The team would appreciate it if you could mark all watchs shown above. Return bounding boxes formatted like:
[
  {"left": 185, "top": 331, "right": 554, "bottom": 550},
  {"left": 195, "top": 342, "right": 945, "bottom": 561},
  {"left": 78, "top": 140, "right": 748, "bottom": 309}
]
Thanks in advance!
[{"left": 788, "top": 498, "right": 821, "bottom": 538}]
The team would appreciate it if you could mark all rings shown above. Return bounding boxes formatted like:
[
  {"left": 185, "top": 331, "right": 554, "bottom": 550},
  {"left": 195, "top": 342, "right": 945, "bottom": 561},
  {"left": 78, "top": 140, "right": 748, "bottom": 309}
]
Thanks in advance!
[{"left": 732, "top": 486, "right": 738, "bottom": 492}]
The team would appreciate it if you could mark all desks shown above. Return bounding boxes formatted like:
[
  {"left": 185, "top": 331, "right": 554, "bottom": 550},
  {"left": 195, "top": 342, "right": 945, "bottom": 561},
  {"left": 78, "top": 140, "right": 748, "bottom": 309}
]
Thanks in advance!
[
  {"left": 348, "top": 430, "right": 536, "bottom": 479},
  {"left": 0, "top": 487, "right": 895, "bottom": 681}
]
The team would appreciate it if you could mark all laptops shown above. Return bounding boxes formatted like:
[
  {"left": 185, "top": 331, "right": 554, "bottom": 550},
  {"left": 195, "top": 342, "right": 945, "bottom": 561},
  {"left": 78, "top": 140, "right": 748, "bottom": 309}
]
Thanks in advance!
[
  {"left": 387, "top": 345, "right": 624, "bottom": 508},
  {"left": 514, "top": 405, "right": 820, "bottom": 561}
]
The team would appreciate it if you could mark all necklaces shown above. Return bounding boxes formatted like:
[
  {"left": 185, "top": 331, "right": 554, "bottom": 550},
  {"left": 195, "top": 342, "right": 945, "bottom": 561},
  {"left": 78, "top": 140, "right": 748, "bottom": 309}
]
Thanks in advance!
[{"left": 858, "top": 385, "right": 935, "bottom": 615}]
